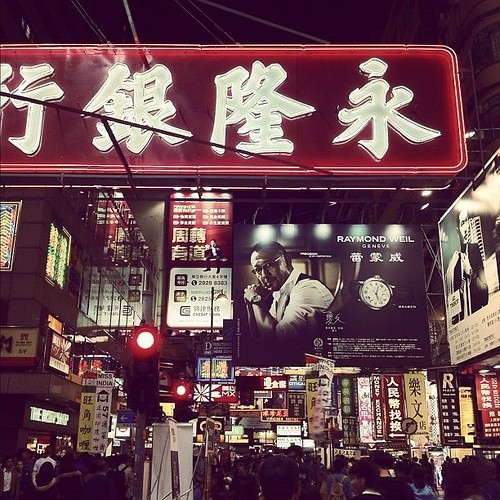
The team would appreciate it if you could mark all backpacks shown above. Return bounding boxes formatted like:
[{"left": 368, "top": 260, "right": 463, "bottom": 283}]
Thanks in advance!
[
  {"left": 329, "top": 474, "right": 347, "bottom": 500},
  {"left": 107, "top": 463, "right": 128, "bottom": 495}
]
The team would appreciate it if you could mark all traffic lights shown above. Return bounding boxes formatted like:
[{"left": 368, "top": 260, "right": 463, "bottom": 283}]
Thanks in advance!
[
  {"left": 174, "top": 382, "right": 188, "bottom": 397},
  {"left": 133, "top": 327, "right": 157, "bottom": 352}
]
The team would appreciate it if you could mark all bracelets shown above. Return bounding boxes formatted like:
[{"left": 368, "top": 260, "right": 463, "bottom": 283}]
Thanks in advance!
[{"left": 470, "top": 272, "right": 479, "bottom": 286}]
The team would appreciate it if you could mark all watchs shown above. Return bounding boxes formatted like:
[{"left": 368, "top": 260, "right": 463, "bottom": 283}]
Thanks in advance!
[
  {"left": 350, "top": 274, "right": 415, "bottom": 312},
  {"left": 249, "top": 293, "right": 263, "bottom": 307}
]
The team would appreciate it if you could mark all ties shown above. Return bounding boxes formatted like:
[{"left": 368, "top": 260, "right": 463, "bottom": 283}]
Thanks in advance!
[{"left": 466, "top": 276, "right": 470, "bottom": 317}]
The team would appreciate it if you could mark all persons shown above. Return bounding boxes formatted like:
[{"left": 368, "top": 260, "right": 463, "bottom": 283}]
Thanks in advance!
[
  {"left": 240, "top": 240, "right": 334, "bottom": 336},
  {"left": 2, "top": 438, "right": 499, "bottom": 500},
  {"left": 449, "top": 205, "right": 487, "bottom": 326},
  {"left": 205, "top": 239, "right": 223, "bottom": 273}
]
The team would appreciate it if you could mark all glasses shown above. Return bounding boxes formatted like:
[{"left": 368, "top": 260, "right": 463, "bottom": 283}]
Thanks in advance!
[{"left": 251, "top": 254, "right": 284, "bottom": 274}]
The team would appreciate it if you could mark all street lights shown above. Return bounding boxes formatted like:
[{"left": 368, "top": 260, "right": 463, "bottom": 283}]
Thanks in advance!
[{"left": 204, "top": 286, "right": 229, "bottom": 500}]
